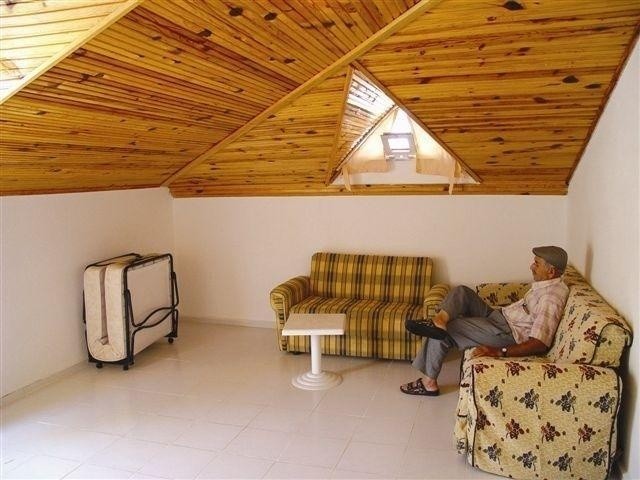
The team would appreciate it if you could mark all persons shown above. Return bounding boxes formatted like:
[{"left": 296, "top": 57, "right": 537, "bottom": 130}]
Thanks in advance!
[{"left": 400, "top": 246, "right": 569, "bottom": 396}]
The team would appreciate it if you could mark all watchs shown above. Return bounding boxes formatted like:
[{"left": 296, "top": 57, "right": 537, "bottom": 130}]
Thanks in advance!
[{"left": 501, "top": 346, "right": 508, "bottom": 357}]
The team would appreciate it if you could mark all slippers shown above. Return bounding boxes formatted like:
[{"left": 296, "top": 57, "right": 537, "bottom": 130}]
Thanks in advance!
[
  {"left": 400, "top": 378, "right": 440, "bottom": 396},
  {"left": 405, "top": 319, "right": 447, "bottom": 340}
]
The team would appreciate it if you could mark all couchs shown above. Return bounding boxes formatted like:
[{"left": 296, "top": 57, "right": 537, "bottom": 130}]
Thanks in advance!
[
  {"left": 268, "top": 251, "right": 452, "bottom": 365},
  {"left": 452, "top": 265, "right": 634, "bottom": 479}
]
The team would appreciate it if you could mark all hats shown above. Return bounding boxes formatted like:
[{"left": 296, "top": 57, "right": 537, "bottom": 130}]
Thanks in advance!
[{"left": 532, "top": 245, "right": 567, "bottom": 271}]
